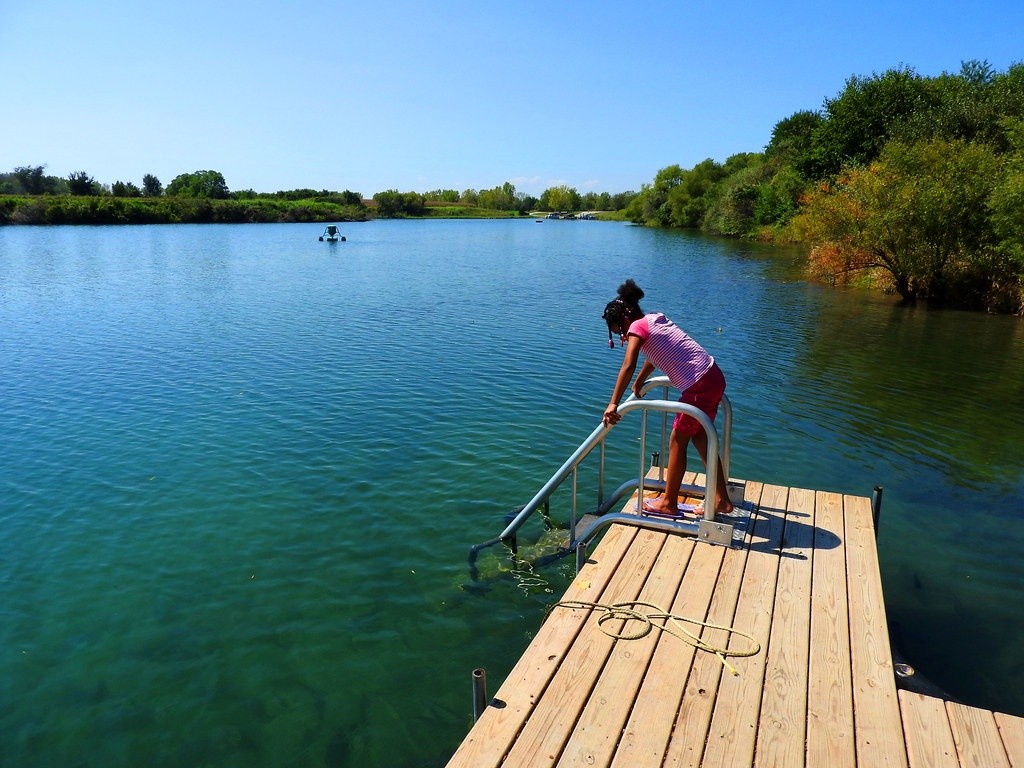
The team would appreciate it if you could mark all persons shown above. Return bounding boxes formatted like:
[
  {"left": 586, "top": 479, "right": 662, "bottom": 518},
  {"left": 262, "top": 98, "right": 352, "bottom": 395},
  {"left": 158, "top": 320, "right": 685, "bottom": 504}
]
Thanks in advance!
[{"left": 603, "top": 279, "right": 734, "bottom": 518}]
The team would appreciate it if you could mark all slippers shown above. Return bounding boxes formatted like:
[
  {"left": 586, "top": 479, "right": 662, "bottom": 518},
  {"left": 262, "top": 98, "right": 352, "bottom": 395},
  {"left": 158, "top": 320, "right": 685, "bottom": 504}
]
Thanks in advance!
[
  {"left": 648, "top": 496, "right": 696, "bottom": 511},
  {"left": 633, "top": 498, "right": 684, "bottom": 518}
]
